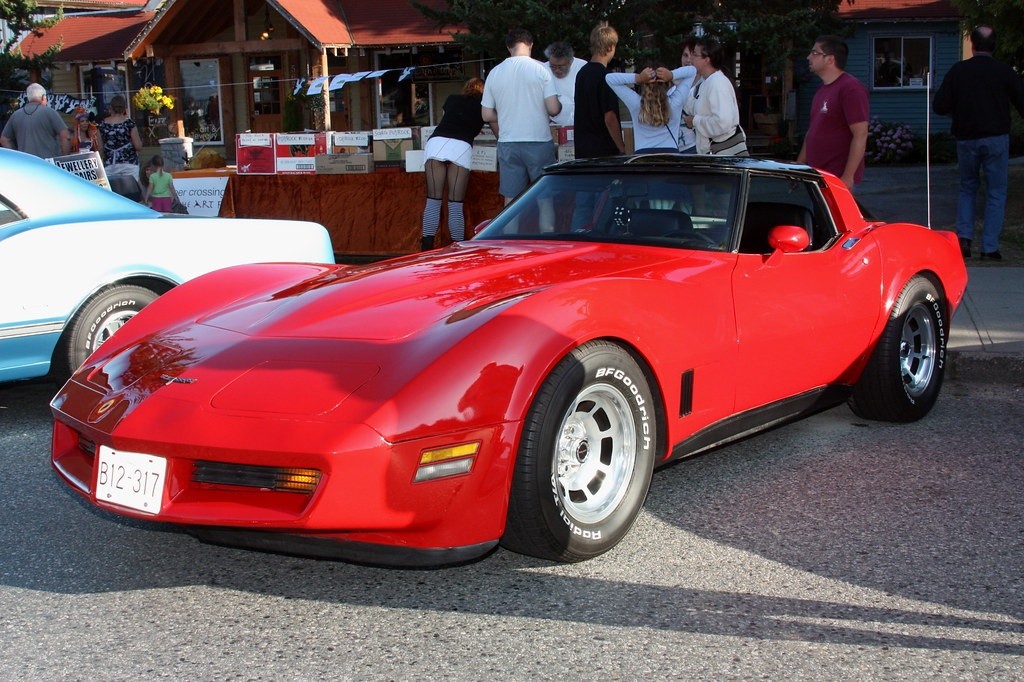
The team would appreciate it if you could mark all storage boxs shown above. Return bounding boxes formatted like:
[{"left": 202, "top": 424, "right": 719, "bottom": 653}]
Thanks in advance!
[
  {"left": 235, "top": 121, "right": 634, "bottom": 174},
  {"left": 754, "top": 112, "right": 782, "bottom": 134}
]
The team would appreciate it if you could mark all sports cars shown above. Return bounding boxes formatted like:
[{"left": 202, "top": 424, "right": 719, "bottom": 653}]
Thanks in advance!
[{"left": 49, "top": 151, "right": 970, "bottom": 572}]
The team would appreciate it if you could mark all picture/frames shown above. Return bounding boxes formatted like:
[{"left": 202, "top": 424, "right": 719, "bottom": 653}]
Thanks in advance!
[{"left": 410, "top": 49, "right": 465, "bottom": 83}]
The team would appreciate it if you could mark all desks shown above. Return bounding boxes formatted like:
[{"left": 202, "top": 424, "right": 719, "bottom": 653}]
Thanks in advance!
[
  {"left": 170, "top": 169, "right": 579, "bottom": 256},
  {"left": 749, "top": 93, "right": 783, "bottom": 129}
]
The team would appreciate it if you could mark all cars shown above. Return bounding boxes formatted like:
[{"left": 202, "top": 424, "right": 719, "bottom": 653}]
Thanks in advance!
[{"left": 0, "top": 148, "right": 335, "bottom": 399}]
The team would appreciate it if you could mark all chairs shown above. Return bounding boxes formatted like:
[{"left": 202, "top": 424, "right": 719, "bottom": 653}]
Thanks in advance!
[
  {"left": 744, "top": 200, "right": 814, "bottom": 253},
  {"left": 628, "top": 209, "right": 695, "bottom": 237}
]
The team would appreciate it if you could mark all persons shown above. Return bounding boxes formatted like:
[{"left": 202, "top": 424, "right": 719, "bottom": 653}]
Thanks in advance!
[
  {"left": 481, "top": 28, "right": 562, "bottom": 234},
  {"left": 796, "top": 33, "right": 870, "bottom": 186},
  {"left": 569, "top": 23, "right": 625, "bottom": 231},
  {"left": 605, "top": 61, "right": 697, "bottom": 152},
  {"left": 146, "top": 155, "right": 180, "bottom": 212},
  {"left": 677, "top": 36, "right": 705, "bottom": 215},
  {"left": 541, "top": 42, "right": 588, "bottom": 126},
  {"left": 0, "top": 83, "right": 70, "bottom": 159},
  {"left": 421, "top": 79, "right": 498, "bottom": 251},
  {"left": 669, "top": 34, "right": 750, "bottom": 215},
  {"left": 70, "top": 108, "right": 102, "bottom": 152},
  {"left": 96, "top": 95, "right": 142, "bottom": 166},
  {"left": 932, "top": 25, "right": 1024, "bottom": 260}
]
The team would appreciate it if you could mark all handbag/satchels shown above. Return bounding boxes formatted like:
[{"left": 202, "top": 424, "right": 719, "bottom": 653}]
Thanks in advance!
[{"left": 708, "top": 124, "right": 750, "bottom": 160}]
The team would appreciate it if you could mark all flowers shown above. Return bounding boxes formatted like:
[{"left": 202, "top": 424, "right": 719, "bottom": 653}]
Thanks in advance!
[{"left": 135, "top": 84, "right": 176, "bottom": 116}]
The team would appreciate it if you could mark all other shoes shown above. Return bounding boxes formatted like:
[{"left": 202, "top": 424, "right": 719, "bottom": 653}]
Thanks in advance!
[
  {"left": 980, "top": 251, "right": 1002, "bottom": 261},
  {"left": 960, "top": 239, "right": 972, "bottom": 257}
]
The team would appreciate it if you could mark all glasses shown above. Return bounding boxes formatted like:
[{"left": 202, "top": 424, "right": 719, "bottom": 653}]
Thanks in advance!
[
  {"left": 809, "top": 48, "right": 827, "bottom": 57},
  {"left": 549, "top": 59, "right": 570, "bottom": 71},
  {"left": 690, "top": 50, "right": 702, "bottom": 59}
]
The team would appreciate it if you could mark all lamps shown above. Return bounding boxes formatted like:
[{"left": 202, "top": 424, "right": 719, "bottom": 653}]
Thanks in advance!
[
  {"left": 412, "top": 46, "right": 417, "bottom": 54},
  {"left": 111, "top": 60, "right": 116, "bottom": 68},
  {"left": 258, "top": 6, "right": 275, "bottom": 41},
  {"left": 45, "top": 67, "right": 50, "bottom": 72},
  {"left": 132, "top": 59, "right": 137, "bottom": 67},
  {"left": 89, "top": 62, "right": 93, "bottom": 69},
  {"left": 385, "top": 47, "right": 391, "bottom": 54},
  {"left": 359, "top": 48, "right": 366, "bottom": 56},
  {"left": 66, "top": 63, "right": 71, "bottom": 71},
  {"left": 439, "top": 45, "right": 443, "bottom": 53}
]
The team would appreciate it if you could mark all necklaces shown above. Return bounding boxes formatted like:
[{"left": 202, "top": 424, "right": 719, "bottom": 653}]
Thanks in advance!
[{"left": 24, "top": 103, "right": 41, "bottom": 115}]
